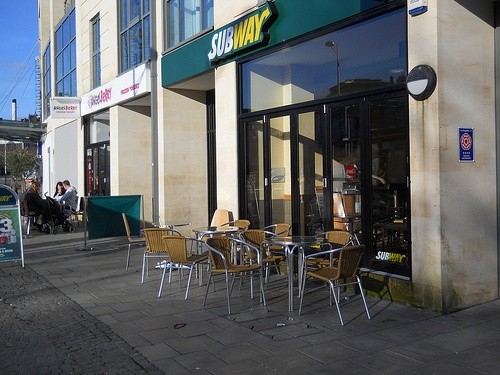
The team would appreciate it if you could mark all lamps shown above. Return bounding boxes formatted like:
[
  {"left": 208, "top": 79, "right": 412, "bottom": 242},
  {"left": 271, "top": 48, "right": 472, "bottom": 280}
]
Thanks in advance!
[{"left": 405, "top": 64, "right": 437, "bottom": 101}]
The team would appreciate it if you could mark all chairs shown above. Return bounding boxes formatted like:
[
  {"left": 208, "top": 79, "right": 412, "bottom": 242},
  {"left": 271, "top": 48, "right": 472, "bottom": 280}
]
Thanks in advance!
[
  {"left": 121, "top": 206, "right": 408, "bottom": 326},
  {"left": 18, "top": 193, "right": 89, "bottom": 237}
]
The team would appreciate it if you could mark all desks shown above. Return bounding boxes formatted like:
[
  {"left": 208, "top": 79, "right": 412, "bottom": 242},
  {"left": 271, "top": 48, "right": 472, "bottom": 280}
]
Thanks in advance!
[
  {"left": 152, "top": 220, "right": 192, "bottom": 274},
  {"left": 192, "top": 226, "right": 244, "bottom": 287},
  {"left": 383, "top": 221, "right": 408, "bottom": 249},
  {"left": 260, "top": 236, "right": 334, "bottom": 311}
]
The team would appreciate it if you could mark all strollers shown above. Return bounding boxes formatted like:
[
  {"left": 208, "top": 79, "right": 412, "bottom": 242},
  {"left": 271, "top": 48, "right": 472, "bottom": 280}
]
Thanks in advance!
[{"left": 40, "top": 191, "right": 73, "bottom": 235}]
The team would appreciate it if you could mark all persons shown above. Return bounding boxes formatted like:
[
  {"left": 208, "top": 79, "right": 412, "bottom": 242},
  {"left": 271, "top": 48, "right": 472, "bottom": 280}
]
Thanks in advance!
[
  {"left": 23, "top": 182, "right": 48, "bottom": 225},
  {"left": 53, "top": 182, "right": 66, "bottom": 203},
  {"left": 54, "top": 180, "right": 77, "bottom": 218}
]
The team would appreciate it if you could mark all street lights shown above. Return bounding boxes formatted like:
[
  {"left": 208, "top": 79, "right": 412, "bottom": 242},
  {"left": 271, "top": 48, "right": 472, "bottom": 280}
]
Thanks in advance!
[{"left": 324, "top": 41, "right": 341, "bottom": 96}]
